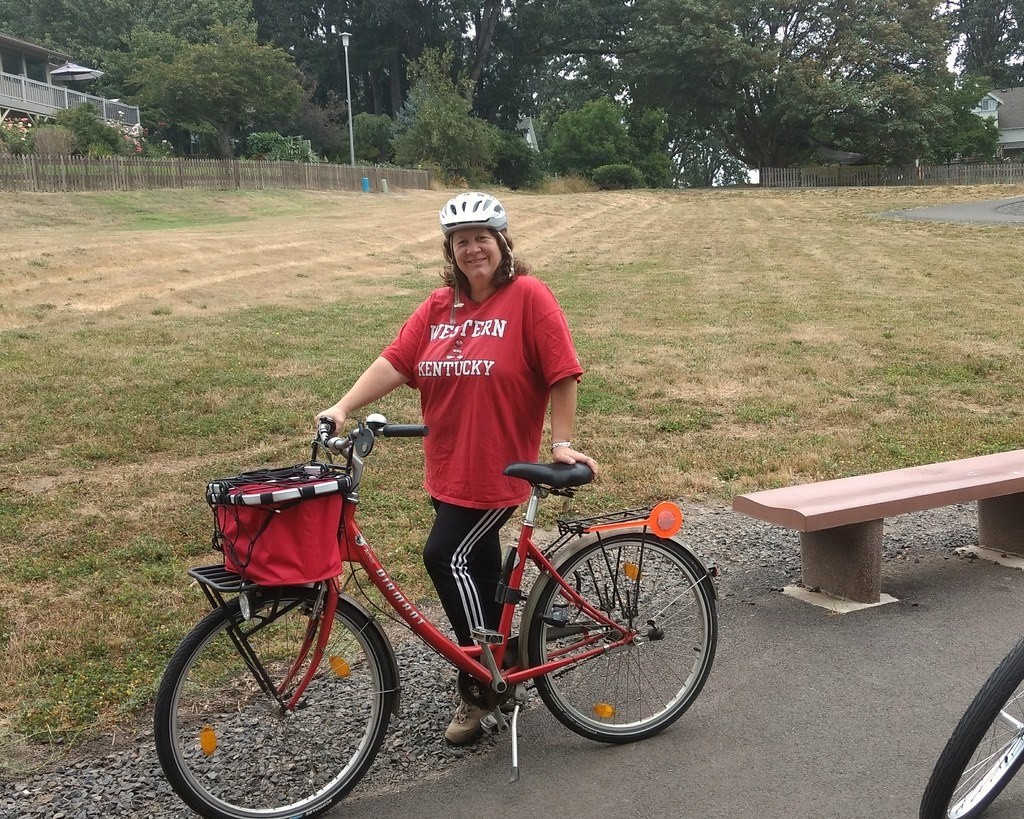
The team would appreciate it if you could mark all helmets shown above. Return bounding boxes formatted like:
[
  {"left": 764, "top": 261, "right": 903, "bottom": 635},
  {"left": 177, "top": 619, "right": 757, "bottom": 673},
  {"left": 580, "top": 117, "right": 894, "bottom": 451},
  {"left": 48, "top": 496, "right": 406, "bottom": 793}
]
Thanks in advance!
[{"left": 439, "top": 192, "right": 508, "bottom": 238}]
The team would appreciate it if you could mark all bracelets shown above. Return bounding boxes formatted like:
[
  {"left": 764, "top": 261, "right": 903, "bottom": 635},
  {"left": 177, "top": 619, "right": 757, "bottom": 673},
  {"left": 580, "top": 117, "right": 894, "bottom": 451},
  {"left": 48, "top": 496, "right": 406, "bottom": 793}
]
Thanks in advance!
[{"left": 550, "top": 442, "right": 572, "bottom": 453}]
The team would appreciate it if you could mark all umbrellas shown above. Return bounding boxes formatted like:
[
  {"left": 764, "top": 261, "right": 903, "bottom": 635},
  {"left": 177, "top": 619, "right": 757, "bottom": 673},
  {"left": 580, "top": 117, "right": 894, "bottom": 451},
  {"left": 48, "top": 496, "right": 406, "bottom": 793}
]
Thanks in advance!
[{"left": 50, "top": 60, "right": 105, "bottom": 90}]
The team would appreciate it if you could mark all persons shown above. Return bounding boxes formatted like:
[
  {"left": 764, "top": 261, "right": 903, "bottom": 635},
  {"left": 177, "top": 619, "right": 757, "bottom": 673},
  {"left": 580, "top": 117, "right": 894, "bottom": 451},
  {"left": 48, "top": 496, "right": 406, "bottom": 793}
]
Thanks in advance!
[{"left": 313, "top": 191, "right": 598, "bottom": 746}]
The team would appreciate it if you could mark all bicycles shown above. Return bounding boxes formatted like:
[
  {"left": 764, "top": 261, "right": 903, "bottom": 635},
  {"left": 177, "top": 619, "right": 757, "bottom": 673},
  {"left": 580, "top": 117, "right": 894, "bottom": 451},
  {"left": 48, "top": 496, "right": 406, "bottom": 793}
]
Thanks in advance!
[
  {"left": 154, "top": 414, "right": 722, "bottom": 819},
  {"left": 918, "top": 637, "right": 1024, "bottom": 819}
]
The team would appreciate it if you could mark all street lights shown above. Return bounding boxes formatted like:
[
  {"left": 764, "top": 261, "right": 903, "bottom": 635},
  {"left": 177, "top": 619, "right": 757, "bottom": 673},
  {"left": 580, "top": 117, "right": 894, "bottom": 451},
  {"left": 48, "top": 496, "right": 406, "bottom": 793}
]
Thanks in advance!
[{"left": 339, "top": 31, "right": 355, "bottom": 166}]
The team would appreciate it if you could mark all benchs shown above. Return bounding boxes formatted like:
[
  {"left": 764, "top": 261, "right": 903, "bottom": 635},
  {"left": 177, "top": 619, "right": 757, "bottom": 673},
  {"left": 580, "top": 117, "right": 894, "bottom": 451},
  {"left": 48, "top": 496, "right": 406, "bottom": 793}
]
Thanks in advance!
[{"left": 733, "top": 449, "right": 1024, "bottom": 604}]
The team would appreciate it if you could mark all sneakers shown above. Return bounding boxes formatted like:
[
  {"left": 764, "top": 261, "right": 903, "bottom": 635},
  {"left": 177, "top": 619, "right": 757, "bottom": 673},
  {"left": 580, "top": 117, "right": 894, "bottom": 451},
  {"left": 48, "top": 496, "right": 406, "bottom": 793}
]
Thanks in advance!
[{"left": 445, "top": 691, "right": 490, "bottom": 743}]
